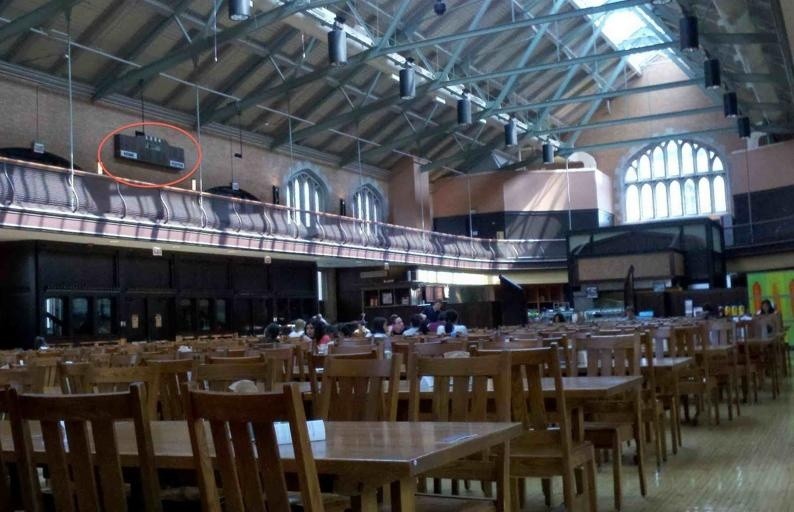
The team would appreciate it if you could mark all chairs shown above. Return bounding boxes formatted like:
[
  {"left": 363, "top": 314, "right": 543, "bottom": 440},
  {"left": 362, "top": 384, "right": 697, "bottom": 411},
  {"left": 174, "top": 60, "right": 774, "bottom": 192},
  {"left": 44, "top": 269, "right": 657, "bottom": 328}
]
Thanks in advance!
[
  {"left": 1, "top": 311, "right": 792, "bottom": 472},
  {"left": 178, "top": 382, "right": 351, "bottom": 511},
  {"left": 404, "top": 349, "right": 515, "bottom": 510},
  {"left": 474, "top": 340, "right": 601, "bottom": 511},
  {"left": 314, "top": 352, "right": 402, "bottom": 510},
  {"left": 2, "top": 381, "right": 165, "bottom": 510},
  {"left": 569, "top": 332, "right": 649, "bottom": 511}
]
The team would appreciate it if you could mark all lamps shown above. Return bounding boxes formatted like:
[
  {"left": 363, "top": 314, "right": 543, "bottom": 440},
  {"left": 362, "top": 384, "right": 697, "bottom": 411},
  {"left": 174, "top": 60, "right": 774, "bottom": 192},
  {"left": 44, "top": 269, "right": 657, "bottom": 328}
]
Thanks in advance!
[
  {"left": 227, "top": 1, "right": 578, "bottom": 167},
  {"left": 649, "top": 1, "right": 762, "bottom": 142}
]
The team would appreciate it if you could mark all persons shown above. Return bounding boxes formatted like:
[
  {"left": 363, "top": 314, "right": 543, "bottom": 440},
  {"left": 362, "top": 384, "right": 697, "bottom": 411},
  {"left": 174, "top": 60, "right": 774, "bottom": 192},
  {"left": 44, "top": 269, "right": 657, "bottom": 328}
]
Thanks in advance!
[
  {"left": 263, "top": 299, "right": 468, "bottom": 350},
  {"left": 550, "top": 313, "right": 565, "bottom": 323},
  {"left": 703, "top": 300, "right": 774, "bottom": 319},
  {"left": 621, "top": 306, "right": 638, "bottom": 321}
]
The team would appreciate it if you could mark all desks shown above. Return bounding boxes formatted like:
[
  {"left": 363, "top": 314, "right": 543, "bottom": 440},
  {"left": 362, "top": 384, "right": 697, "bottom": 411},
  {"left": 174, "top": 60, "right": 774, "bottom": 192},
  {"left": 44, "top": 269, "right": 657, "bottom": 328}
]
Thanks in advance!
[
  {"left": 350, "top": 375, "right": 649, "bottom": 510},
  {"left": 2, "top": 419, "right": 522, "bottom": 511}
]
